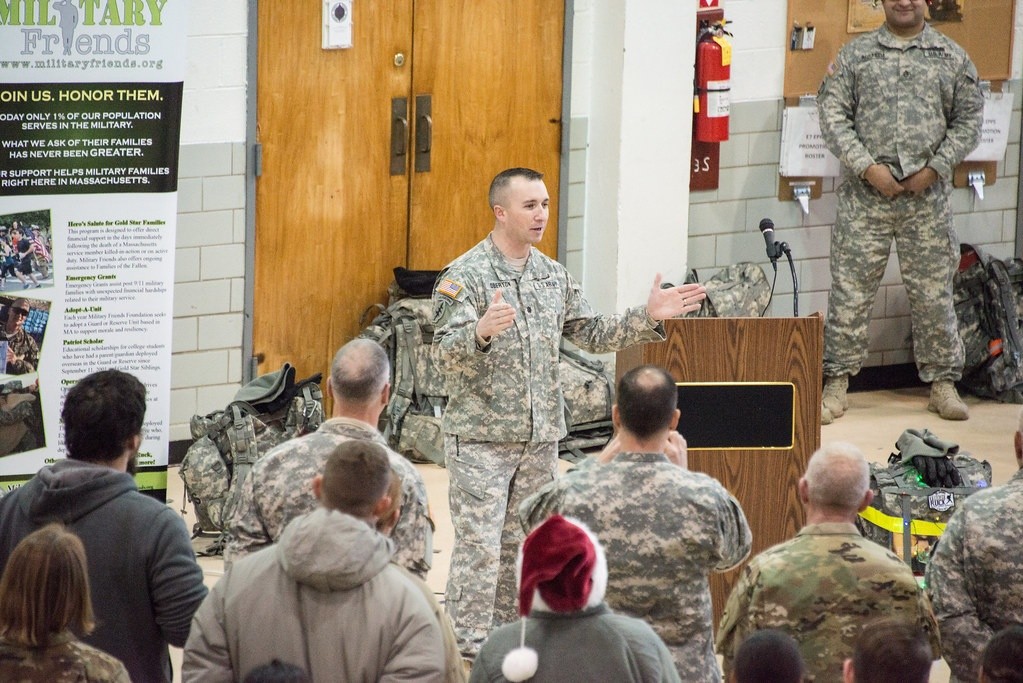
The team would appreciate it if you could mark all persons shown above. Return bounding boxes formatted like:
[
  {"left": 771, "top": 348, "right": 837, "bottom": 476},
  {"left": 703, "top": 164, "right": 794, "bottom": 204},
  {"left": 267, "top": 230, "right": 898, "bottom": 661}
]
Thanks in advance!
[
  {"left": 730, "top": 630, "right": 803, "bottom": 683},
  {"left": 0, "top": 297, "right": 39, "bottom": 373},
  {"left": 817, "top": 0, "right": 985, "bottom": 425},
  {"left": 0, "top": 222, "right": 48, "bottom": 291},
  {"left": 468, "top": 514, "right": 680, "bottom": 683},
  {"left": 843, "top": 615, "right": 934, "bottom": 683},
  {"left": 0, "top": 520, "right": 132, "bottom": 683},
  {"left": 927, "top": 412, "right": 1023, "bottom": 683},
  {"left": 181, "top": 440, "right": 468, "bottom": 683},
  {"left": 0, "top": 380, "right": 45, "bottom": 454},
  {"left": 716, "top": 442, "right": 942, "bottom": 683},
  {"left": 0, "top": 368, "right": 209, "bottom": 683},
  {"left": 521, "top": 365, "right": 753, "bottom": 683},
  {"left": 431, "top": 167, "right": 706, "bottom": 671},
  {"left": 223, "top": 339, "right": 436, "bottom": 580}
]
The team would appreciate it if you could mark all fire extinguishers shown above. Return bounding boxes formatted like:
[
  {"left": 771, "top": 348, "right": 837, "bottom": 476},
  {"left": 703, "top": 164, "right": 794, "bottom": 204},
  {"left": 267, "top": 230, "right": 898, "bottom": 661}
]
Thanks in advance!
[{"left": 694, "top": 20, "right": 732, "bottom": 142}]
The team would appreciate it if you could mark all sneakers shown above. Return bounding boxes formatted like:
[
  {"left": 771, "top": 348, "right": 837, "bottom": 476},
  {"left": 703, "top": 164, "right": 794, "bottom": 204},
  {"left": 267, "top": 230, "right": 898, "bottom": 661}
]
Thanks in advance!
[
  {"left": 820, "top": 374, "right": 849, "bottom": 425},
  {"left": 927, "top": 379, "right": 970, "bottom": 421}
]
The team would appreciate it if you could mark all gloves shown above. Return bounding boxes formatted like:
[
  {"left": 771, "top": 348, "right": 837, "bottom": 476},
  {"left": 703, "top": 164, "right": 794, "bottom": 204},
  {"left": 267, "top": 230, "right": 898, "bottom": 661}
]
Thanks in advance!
[{"left": 894, "top": 442, "right": 961, "bottom": 488}]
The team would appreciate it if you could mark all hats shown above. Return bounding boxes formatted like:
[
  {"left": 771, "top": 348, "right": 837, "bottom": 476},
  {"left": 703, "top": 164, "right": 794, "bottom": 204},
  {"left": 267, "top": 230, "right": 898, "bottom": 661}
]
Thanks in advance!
[
  {"left": 2, "top": 380, "right": 22, "bottom": 394},
  {"left": 501, "top": 515, "right": 608, "bottom": 683}
]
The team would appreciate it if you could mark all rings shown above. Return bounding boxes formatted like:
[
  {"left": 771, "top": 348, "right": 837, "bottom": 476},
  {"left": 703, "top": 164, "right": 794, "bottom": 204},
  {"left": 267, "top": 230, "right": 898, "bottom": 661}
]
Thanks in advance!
[{"left": 684, "top": 299, "right": 687, "bottom": 305}]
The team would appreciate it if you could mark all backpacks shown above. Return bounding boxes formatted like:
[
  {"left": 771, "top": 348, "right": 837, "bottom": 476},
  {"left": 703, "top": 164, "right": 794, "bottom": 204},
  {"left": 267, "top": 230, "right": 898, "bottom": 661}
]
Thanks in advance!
[
  {"left": 855, "top": 453, "right": 992, "bottom": 577},
  {"left": 660, "top": 261, "right": 772, "bottom": 318},
  {"left": 951, "top": 243, "right": 1023, "bottom": 405},
  {"left": 557, "top": 349, "right": 617, "bottom": 464},
  {"left": 356, "top": 281, "right": 449, "bottom": 469},
  {"left": 178, "top": 382, "right": 323, "bottom": 558}
]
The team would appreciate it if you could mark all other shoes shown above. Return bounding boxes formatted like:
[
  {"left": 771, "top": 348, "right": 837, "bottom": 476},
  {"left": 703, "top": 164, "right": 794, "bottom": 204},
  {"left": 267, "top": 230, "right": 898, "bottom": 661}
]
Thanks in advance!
[
  {"left": 36, "top": 285, "right": 41, "bottom": 288},
  {"left": 23, "top": 284, "right": 31, "bottom": 290}
]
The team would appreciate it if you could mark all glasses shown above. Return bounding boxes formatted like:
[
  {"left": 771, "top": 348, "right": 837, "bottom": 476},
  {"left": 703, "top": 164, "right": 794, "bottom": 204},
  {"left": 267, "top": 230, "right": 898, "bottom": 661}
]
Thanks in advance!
[{"left": 11, "top": 307, "right": 29, "bottom": 317}]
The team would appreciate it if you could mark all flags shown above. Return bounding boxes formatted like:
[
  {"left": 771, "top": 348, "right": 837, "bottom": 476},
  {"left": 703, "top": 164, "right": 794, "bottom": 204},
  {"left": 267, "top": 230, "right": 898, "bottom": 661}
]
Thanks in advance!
[{"left": 22, "top": 225, "right": 52, "bottom": 262}]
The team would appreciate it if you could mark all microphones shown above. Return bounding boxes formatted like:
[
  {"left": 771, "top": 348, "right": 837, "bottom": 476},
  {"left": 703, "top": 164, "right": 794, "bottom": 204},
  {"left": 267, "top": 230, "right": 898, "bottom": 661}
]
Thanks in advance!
[{"left": 759, "top": 218, "right": 777, "bottom": 271}]
